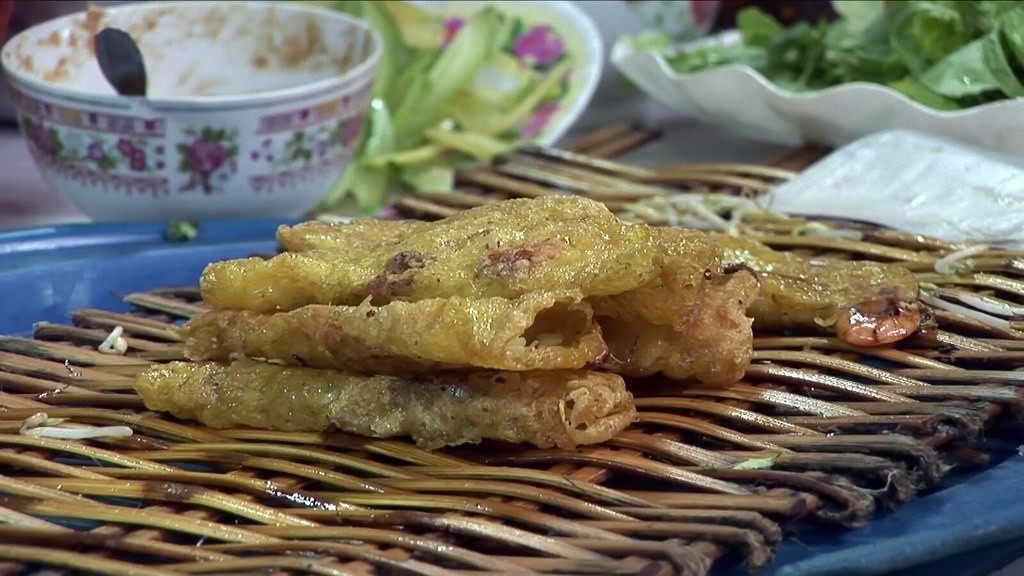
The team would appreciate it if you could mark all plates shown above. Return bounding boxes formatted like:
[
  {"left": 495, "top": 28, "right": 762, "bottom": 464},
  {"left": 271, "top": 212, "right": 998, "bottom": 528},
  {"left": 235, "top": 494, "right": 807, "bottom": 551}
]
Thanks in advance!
[
  {"left": 610, "top": 30, "right": 1023, "bottom": 157},
  {"left": 2, "top": 221, "right": 1024, "bottom": 576},
  {"left": 1, "top": 219, "right": 1024, "bottom": 575},
  {"left": 382, "top": 0, "right": 604, "bottom": 149}
]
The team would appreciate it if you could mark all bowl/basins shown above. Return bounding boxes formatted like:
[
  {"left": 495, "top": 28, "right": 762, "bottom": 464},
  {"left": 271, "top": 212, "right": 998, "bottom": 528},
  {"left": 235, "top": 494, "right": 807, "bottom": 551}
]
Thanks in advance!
[{"left": 0, "top": 1, "right": 386, "bottom": 220}]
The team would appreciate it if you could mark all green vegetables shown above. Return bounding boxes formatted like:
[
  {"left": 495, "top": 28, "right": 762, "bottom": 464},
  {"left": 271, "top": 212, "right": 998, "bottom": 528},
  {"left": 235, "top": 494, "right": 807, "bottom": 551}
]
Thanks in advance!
[
  {"left": 634, "top": 0, "right": 1024, "bottom": 116},
  {"left": 292, "top": 1, "right": 567, "bottom": 206}
]
